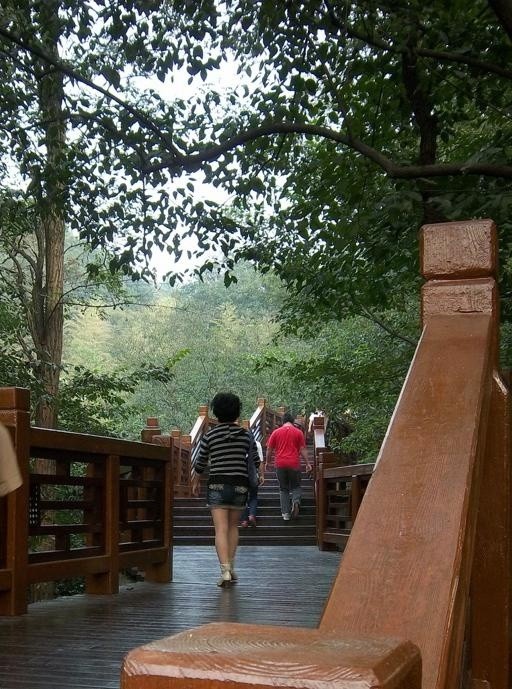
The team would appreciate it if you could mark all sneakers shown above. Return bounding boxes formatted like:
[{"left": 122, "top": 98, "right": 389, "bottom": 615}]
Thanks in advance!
[
  {"left": 282, "top": 501, "right": 300, "bottom": 520},
  {"left": 241, "top": 515, "right": 256, "bottom": 528}
]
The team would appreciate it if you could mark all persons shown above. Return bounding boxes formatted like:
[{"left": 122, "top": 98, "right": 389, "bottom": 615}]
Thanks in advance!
[
  {"left": 264, "top": 413, "right": 313, "bottom": 521},
  {"left": 241, "top": 441, "right": 266, "bottom": 528},
  {"left": 189, "top": 391, "right": 261, "bottom": 588},
  {"left": 308, "top": 411, "right": 320, "bottom": 432}
]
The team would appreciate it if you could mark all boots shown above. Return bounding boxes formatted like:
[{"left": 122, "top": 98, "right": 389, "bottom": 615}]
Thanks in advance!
[{"left": 217, "top": 558, "right": 237, "bottom": 588}]
